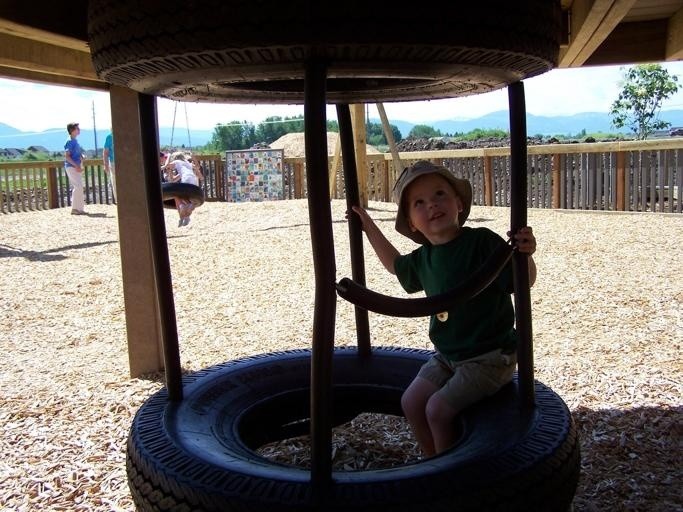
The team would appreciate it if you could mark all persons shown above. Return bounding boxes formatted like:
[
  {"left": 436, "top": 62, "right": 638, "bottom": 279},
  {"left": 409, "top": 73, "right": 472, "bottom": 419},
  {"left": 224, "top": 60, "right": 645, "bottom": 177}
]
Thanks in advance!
[
  {"left": 64, "top": 123, "right": 88, "bottom": 216},
  {"left": 103, "top": 132, "right": 117, "bottom": 204},
  {"left": 160, "top": 151, "right": 203, "bottom": 228},
  {"left": 346, "top": 161, "right": 537, "bottom": 457}
]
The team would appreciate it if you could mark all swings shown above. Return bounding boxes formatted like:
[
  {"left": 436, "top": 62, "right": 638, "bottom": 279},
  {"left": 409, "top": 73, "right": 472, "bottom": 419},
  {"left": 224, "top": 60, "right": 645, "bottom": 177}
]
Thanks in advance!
[{"left": 161, "top": 101, "right": 204, "bottom": 210}]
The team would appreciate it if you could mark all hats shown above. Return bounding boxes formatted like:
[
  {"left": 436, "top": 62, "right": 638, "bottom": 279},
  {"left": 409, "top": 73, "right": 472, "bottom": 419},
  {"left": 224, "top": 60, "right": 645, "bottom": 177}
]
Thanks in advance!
[{"left": 394, "top": 160, "right": 473, "bottom": 245}]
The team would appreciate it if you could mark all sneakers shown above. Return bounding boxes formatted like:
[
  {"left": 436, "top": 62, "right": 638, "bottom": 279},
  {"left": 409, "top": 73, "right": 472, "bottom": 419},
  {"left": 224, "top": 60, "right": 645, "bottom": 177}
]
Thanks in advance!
[{"left": 72, "top": 208, "right": 89, "bottom": 215}]
